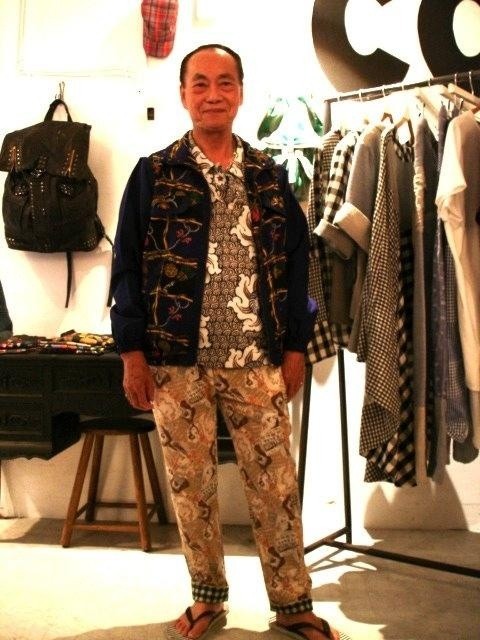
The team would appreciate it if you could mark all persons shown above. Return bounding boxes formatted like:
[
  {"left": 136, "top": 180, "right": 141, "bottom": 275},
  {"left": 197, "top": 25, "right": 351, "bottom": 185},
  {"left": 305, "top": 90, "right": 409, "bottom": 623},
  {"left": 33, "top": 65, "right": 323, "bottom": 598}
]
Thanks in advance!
[{"left": 105, "top": 41, "right": 342, "bottom": 640}]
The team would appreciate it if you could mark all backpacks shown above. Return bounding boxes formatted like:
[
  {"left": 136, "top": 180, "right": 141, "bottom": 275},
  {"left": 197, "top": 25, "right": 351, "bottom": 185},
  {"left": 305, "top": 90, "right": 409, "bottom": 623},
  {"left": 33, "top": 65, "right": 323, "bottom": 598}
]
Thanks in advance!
[{"left": 1, "top": 99, "right": 107, "bottom": 253}]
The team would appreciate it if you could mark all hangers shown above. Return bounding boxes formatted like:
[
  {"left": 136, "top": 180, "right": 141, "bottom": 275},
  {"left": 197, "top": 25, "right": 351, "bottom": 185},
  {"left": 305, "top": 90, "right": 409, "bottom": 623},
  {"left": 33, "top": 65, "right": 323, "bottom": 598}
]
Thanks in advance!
[{"left": 329, "top": 68, "right": 480, "bottom": 145}]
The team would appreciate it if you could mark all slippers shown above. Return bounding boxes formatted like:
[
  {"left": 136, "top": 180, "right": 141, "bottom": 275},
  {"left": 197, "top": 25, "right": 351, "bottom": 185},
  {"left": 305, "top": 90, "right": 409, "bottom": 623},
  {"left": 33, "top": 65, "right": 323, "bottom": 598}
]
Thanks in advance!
[
  {"left": 163, "top": 603, "right": 230, "bottom": 640},
  {"left": 267, "top": 610, "right": 351, "bottom": 640}
]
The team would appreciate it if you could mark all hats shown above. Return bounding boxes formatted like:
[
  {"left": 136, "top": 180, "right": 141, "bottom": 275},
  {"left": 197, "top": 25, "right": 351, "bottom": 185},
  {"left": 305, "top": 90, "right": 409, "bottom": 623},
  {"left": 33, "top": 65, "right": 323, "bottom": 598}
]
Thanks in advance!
[{"left": 140, "top": 0, "right": 181, "bottom": 58}]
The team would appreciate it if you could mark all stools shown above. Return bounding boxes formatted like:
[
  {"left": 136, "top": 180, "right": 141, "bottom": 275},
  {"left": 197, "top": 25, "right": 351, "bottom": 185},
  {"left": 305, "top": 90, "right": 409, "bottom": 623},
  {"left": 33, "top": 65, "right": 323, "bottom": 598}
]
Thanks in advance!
[{"left": 60, "top": 418, "right": 168, "bottom": 553}]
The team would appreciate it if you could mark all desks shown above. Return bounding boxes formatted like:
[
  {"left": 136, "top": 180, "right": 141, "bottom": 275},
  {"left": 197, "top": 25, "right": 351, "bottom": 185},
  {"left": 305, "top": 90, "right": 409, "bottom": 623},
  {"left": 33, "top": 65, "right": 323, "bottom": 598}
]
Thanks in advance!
[{"left": 0, "top": 347, "right": 238, "bottom": 466}]
